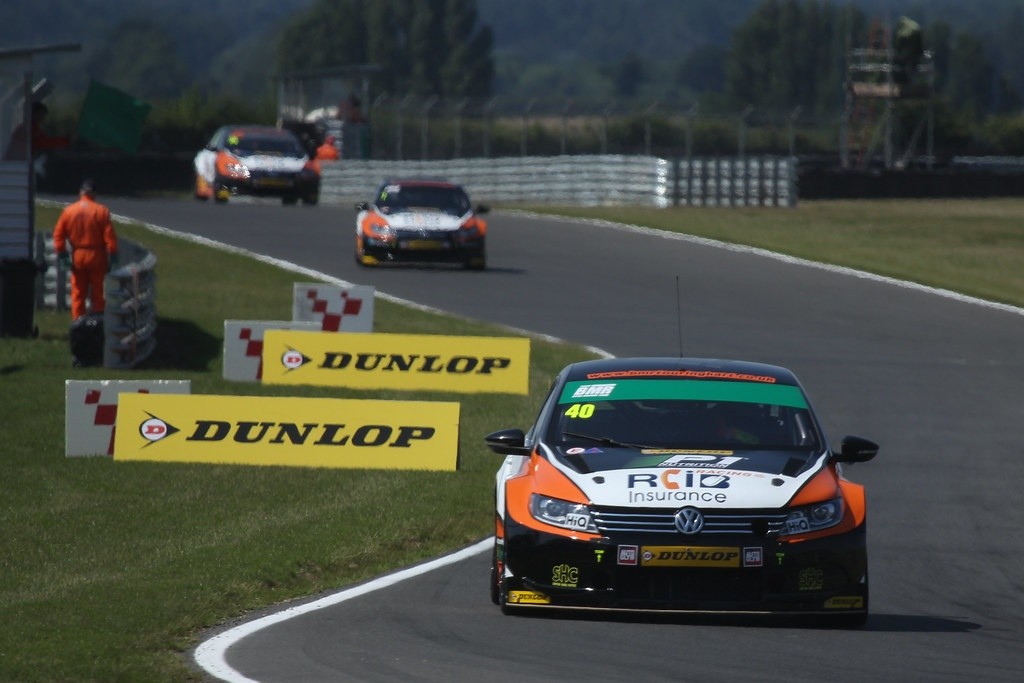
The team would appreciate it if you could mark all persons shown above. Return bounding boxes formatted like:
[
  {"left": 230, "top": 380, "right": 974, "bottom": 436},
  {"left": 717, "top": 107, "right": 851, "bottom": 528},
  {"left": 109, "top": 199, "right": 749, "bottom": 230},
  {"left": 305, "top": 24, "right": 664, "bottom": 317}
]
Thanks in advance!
[
  {"left": 51, "top": 179, "right": 115, "bottom": 326},
  {"left": 702, "top": 403, "right": 760, "bottom": 447},
  {"left": 309, "top": 136, "right": 339, "bottom": 177}
]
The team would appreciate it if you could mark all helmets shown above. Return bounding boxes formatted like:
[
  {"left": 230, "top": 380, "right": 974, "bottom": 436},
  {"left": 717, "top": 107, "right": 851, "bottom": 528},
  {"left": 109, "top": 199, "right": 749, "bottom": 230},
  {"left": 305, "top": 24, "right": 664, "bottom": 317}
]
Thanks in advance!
[{"left": 711, "top": 403, "right": 760, "bottom": 445}]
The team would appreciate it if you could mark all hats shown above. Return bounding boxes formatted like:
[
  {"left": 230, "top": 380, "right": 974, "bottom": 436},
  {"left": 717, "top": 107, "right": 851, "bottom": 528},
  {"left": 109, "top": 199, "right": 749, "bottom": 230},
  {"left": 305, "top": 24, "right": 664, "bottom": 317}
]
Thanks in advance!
[
  {"left": 81, "top": 180, "right": 96, "bottom": 192},
  {"left": 326, "top": 136, "right": 335, "bottom": 144}
]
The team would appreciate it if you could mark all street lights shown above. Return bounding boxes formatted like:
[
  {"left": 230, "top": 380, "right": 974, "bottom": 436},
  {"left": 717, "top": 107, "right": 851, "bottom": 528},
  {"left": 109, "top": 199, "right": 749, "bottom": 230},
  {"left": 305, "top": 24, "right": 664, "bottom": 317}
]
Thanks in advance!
[{"left": 29, "top": 77, "right": 56, "bottom": 331}]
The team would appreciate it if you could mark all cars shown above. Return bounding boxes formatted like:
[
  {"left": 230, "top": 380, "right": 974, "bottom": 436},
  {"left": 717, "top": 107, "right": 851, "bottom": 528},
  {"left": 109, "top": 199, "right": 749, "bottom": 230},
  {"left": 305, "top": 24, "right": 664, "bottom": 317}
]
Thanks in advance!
[
  {"left": 353, "top": 181, "right": 491, "bottom": 273},
  {"left": 481, "top": 357, "right": 878, "bottom": 628},
  {"left": 194, "top": 125, "right": 324, "bottom": 206}
]
